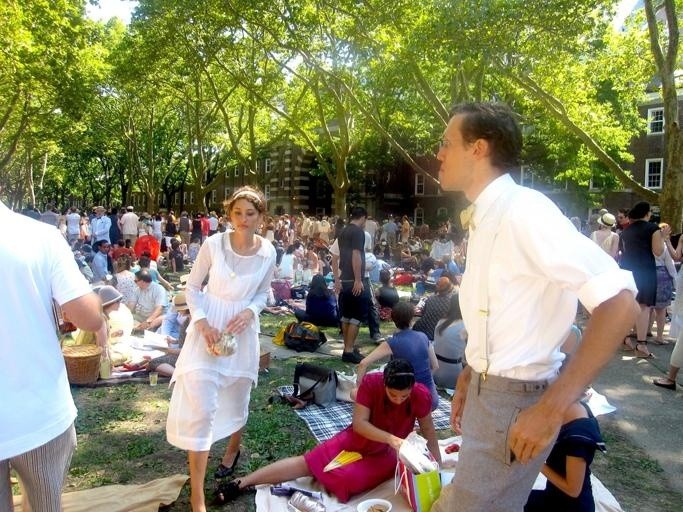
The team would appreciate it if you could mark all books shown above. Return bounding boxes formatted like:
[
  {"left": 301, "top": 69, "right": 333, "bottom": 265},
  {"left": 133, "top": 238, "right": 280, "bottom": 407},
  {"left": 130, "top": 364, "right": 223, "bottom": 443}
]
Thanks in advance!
[{"left": 143, "top": 328, "right": 169, "bottom": 350}]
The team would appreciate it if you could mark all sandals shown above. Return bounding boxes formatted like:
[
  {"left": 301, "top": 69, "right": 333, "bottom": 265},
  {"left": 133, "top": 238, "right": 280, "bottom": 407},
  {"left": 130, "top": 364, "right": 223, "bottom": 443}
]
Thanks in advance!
[{"left": 214, "top": 480, "right": 242, "bottom": 505}]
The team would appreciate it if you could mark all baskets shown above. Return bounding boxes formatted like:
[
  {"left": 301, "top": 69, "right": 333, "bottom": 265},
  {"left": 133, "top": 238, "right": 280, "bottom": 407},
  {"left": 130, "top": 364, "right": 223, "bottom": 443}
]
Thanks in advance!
[{"left": 62, "top": 344, "right": 103, "bottom": 385}]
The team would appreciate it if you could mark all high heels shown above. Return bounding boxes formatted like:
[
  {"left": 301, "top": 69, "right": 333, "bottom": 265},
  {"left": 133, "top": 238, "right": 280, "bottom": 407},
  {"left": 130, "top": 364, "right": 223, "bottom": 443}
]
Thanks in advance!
[
  {"left": 287, "top": 396, "right": 307, "bottom": 409},
  {"left": 634, "top": 340, "right": 656, "bottom": 360},
  {"left": 623, "top": 335, "right": 634, "bottom": 351},
  {"left": 213, "top": 450, "right": 240, "bottom": 478},
  {"left": 298, "top": 391, "right": 312, "bottom": 406}
]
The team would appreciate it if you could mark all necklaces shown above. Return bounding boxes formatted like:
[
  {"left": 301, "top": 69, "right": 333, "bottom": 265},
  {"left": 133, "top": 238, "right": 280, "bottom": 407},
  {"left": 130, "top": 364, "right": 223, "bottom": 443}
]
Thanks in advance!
[{"left": 229, "top": 244, "right": 251, "bottom": 278}]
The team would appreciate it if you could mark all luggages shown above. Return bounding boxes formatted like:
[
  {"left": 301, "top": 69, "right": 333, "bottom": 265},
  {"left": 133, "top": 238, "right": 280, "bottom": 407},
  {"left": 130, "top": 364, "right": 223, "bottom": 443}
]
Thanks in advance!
[{"left": 161, "top": 233, "right": 167, "bottom": 252}]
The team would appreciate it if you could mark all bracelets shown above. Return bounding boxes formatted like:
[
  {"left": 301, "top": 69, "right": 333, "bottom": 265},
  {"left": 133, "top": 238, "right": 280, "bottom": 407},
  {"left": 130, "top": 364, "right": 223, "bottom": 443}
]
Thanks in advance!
[{"left": 545, "top": 467, "right": 553, "bottom": 480}]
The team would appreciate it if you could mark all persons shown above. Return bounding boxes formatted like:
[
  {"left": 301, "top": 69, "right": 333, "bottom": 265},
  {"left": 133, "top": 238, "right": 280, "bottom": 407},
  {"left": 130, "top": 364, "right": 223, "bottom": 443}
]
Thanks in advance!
[
  {"left": 431, "top": 293, "right": 469, "bottom": 389},
  {"left": 364, "top": 206, "right": 378, "bottom": 247},
  {"left": 409, "top": 276, "right": 459, "bottom": 342},
  {"left": 132, "top": 256, "right": 174, "bottom": 291},
  {"left": 295, "top": 275, "right": 339, "bottom": 326},
  {"left": 108, "top": 204, "right": 164, "bottom": 259},
  {"left": 215, "top": 358, "right": 442, "bottom": 505},
  {"left": 521, "top": 355, "right": 607, "bottom": 511},
  {"left": 430, "top": 104, "right": 642, "bottom": 510},
  {"left": 165, "top": 185, "right": 277, "bottom": 512},
  {"left": 371, "top": 268, "right": 400, "bottom": 322},
  {"left": 73, "top": 284, "right": 133, "bottom": 379},
  {"left": 350, "top": 301, "right": 439, "bottom": 408},
  {"left": 126, "top": 270, "right": 169, "bottom": 335},
  {"left": 148, "top": 293, "right": 192, "bottom": 377},
  {"left": 378, "top": 212, "right": 462, "bottom": 283},
  {"left": 161, "top": 210, "right": 224, "bottom": 273},
  {"left": 133, "top": 250, "right": 158, "bottom": 271},
  {"left": 0, "top": 195, "right": 103, "bottom": 512},
  {"left": 107, "top": 254, "right": 137, "bottom": 309},
  {"left": 262, "top": 286, "right": 287, "bottom": 313},
  {"left": 274, "top": 209, "right": 347, "bottom": 279},
  {"left": 569, "top": 200, "right": 682, "bottom": 388},
  {"left": 156, "top": 274, "right": 203, "bottom": 351},
  {"left": 330, "top": 209, "right": 386, "bottom": 344},
  {"left": 337, "top": 206, "right": 374, "bottom": 363},
  {"left": 15, "top": 202, "right": 112, "bottom": 281}
]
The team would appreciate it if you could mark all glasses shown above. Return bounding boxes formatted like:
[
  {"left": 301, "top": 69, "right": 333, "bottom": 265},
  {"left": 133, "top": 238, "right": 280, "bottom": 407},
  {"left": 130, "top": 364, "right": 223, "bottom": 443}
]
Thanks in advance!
[
  {"left": 134, "top": 279, "right": 142, "bottom": 284},
  {"left": 439, "top": 136, "right": 475, "bottom": 147}
]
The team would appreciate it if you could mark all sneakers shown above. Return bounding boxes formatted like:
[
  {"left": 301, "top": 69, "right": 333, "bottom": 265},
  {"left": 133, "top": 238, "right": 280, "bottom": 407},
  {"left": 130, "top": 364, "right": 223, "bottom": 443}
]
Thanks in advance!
[
  {"left": 354, "top": 349, "right": 364, "bottom": 359},
  {"left": 370, "top": 333, "right": 385, "bottom": 343},
  {"left": 341, "top": 353, "right": 361, "bottom": 363}
]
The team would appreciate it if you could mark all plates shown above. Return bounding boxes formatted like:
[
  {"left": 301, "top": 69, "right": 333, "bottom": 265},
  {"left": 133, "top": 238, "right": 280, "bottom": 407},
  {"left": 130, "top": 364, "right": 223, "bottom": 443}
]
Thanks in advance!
[{"left": 357, "top": 499, "right": 393, "bottom": 511}]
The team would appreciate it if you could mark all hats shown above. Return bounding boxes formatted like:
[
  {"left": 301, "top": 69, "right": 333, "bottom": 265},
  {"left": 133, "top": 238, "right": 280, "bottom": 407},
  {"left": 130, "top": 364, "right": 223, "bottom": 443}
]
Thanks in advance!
[
  {"left": 170, "top": 293, "right": 189, "bottom": 312},
  {"left": 126, "top": 205, "right": 132, "bottom": 210},
  {"left": 95, "top": 206, "right": 106, "bottom": 213},
  {"left": 434, "top": 277, "right": 454, "bottom": 294},
  {"left": 209, "top": 211, "right": 217, "bottom": 217},
  {"left": 99, "top": 285, "right": 124, "bottom": 307},
  {"left": 142, "top": 211, "right": 151, "bottom": 219},
  {"left": 597, "top": 213, "right": 615, "bottom": 228}
]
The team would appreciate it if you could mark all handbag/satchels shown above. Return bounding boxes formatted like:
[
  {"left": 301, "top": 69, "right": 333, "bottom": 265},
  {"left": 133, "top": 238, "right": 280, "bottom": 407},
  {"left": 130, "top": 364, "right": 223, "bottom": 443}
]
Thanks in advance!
[
  {"left": 394, "top": 458, "right": 442, "bottom": 512},
  {"left": 204, "top": 329, "right": 236, "bottom": 357},
  {"left": 291, "top": 287, "right": 304, "bottom": 299},
  {"left": 291, "top": 361, "right": 338, "bottom": 409},
  {"left": 300, "top": 321, "right": 318, "bottom": 334},
  {"left": 284, "top": 322, "right": 326, "bottom": 353},
  {"left": 272, "top": 326, "right": 285, "bottom": 346},
  {"left": 336, "top": 369, "right": 357, "bottom": 404},
  {"left": 272, "top": 280, "right": 291, "bottom": 300}
]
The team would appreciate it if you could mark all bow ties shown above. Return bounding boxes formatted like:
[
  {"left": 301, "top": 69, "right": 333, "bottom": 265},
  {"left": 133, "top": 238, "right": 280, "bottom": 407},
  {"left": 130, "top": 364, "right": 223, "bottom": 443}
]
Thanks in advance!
[{"left": 97, "top": 217, "right": 100, "bottom": 219}]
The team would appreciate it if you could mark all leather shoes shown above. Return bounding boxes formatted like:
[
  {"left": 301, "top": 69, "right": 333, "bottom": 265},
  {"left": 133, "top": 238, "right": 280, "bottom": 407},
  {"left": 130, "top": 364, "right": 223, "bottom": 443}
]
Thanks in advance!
[
  {"left": 653, "top": 379, "right": 677, "bottom": 391},
  {"left": 655, "top": 340, "right": 668, "bottom": 345}
]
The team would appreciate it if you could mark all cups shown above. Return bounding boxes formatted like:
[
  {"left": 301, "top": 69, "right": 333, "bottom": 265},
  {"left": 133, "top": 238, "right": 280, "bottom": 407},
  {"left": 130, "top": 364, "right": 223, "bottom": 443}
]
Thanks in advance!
[
  {"left": 149, "top": 373, "right": 159, "bottom": 386},
  {"left": 413, "top": 283, "right": 416, "bottom": 290}
]
